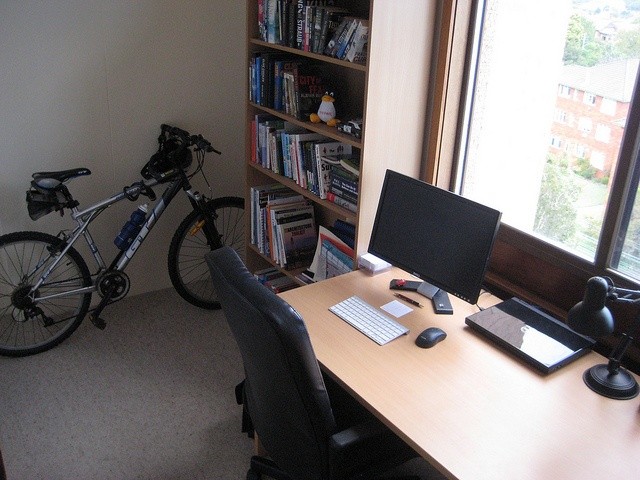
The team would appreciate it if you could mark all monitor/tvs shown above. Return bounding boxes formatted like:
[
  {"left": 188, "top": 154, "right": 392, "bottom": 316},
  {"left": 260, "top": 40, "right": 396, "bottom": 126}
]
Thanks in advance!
[{"left": 367, "top": 169, "right": 504, "bottom": 315}]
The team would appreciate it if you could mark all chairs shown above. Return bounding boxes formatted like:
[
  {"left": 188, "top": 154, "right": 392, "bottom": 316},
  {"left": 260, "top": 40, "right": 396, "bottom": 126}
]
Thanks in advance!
[{"left": 205, "top": 245, "right": 417, "bottom": 480}]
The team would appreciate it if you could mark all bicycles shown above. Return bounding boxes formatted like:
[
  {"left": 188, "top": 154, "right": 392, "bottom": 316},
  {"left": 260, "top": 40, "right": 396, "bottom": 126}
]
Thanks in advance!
[{"left": 0, "top": 124, "right": 245, "bottom": 357}]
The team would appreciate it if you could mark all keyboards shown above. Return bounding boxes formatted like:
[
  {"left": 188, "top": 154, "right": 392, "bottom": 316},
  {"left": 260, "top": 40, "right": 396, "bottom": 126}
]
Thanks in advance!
[{"left": 329, "top": 295, "right": 410, "bottom": 346}]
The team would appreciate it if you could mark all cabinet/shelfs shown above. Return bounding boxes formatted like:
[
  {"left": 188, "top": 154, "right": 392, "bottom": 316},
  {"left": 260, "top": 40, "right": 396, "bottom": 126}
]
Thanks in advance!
[{"left": 244, "top": 1, "right": 438, "bottom": 291}]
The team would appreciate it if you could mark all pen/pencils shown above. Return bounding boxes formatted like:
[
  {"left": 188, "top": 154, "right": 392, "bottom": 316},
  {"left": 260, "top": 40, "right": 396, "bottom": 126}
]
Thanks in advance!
[{"left": 394, "top": 292, "right": 425, "bottom": 308}]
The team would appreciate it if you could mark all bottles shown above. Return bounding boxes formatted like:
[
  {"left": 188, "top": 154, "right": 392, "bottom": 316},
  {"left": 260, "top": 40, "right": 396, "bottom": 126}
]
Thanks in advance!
[{"left": 114, "top": 202, "right": 149, "bottom": 250}]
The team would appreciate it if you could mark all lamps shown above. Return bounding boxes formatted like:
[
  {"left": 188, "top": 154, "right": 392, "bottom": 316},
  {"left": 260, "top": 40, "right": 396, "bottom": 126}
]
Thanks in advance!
[{"left": 566, "top": 276, "right": 639, "bottom": 400}]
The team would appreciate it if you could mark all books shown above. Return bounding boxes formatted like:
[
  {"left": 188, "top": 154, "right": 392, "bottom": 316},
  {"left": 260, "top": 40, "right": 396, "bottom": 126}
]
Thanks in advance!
[
  {"left": 257, "top": 0, "right": 369, "bottom": 63},
  {"left": 250, "top": 112, "right": 360, "bottom": 213},
  {"left": 249, "top": 49, "right": 328, "bottom": 121},
  {"left": 252, "top": 266, "right": 300, "bottom": 296},
  {"left": 251, "top": 180, "right": 318, "bottom": 271},
  {"left": 293, "top": 218, "right": 357, "bottom": 285}
]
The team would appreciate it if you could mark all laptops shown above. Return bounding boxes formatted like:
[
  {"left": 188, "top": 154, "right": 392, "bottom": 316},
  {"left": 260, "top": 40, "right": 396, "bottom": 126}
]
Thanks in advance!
[{"left": 465, "top": 296, "right": 590, "bottom": 374}]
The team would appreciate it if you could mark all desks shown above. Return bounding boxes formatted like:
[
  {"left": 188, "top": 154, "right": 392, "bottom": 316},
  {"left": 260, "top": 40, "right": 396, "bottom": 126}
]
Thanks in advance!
[{"left": 275, "top": 264, "right": 640, "bottom": 480}]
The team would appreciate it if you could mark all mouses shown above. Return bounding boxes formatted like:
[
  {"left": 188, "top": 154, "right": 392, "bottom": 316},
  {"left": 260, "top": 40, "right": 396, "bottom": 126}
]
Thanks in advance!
[{"left": 415, "top": 327, "right": 447, "bottom": 347}]
